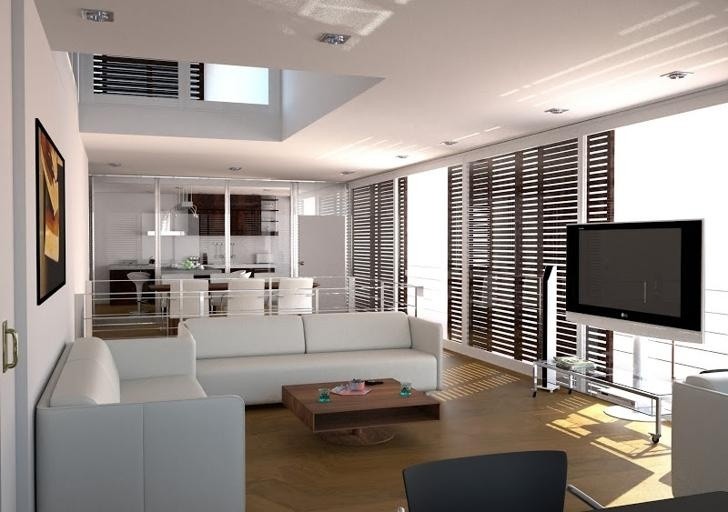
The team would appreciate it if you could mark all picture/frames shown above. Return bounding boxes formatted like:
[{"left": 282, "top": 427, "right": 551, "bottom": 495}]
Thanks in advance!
[{"left": 35, "top": 118, "right": 66, "bottom": 306}]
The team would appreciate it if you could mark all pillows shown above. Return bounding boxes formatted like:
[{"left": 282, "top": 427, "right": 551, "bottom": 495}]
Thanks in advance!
[{"left": 48, "top": 336, "right": 121, "bottom": 406}]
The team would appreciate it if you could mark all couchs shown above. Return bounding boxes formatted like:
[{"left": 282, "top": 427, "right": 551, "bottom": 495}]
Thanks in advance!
[
  {"left": 178, "top": 311, "right": 444, "bottom": 405},
  {"left": 36, "top": 336, "right": 246, "bottom": 512}
]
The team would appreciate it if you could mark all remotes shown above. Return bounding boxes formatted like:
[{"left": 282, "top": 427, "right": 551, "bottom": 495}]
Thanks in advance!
[{"left": 361, "top": 379, "right": 384, "bottom": 385}]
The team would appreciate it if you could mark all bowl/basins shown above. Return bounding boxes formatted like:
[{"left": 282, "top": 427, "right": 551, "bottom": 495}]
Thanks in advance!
[{"left": 348, "top": 381, "right": 365, "bottom": 391}]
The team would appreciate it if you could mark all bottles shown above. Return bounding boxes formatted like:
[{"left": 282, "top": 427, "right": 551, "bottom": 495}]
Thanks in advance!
[{"left": 189, "top": 256, "right": 199, "bottom": 266}]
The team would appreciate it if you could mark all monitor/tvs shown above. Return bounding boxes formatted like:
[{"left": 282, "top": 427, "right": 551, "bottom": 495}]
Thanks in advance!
[{"left": 565, "top": 220, "right": 702, "bottom": 345}]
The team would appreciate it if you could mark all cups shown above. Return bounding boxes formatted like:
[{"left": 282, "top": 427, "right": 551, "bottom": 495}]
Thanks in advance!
[
  {"left": 399, "top": 382, "right": 407, "bottom": 396},
  {"left": 319, "top": 389, "right": 330, "bottom": 403}
]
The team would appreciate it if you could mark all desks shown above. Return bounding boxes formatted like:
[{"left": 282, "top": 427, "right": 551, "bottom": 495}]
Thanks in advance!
[
  {"left": 107, "top": 265, "right": 155, "bottom": 305},
  {"left": 532, "top": 361, "right": 672, "bottom": 444},
  {"left": 582, "top": 490, "right": 728, "bottom": 512}
]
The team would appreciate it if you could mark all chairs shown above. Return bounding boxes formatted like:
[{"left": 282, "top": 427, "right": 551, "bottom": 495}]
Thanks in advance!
[
  {"left": 161, "top": 268, "right": 313, "bottom": 335},
  {"left": 672, "top": 371, "right": 728, "bottom": 498},
  {"left": 398, "top": 450, "right": 605, "bottom": 512}
]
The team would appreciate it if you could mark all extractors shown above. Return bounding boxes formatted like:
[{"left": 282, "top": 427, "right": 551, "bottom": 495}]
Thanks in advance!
[{"left": 146, "top": 211, "right": 185, "bottom": 237}]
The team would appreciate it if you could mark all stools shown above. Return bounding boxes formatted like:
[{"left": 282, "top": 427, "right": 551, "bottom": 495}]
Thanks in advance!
[{"left": 127, "top": 272, "right": 151, "bottom": 313}]
